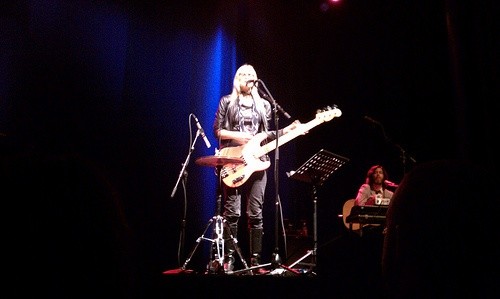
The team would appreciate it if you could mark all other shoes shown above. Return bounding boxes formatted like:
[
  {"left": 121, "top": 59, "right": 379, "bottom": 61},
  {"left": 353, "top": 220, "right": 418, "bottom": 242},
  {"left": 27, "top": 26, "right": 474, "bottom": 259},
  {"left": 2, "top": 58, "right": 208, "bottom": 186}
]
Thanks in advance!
[
  {"left": 249, "top": 258, "right": 270, "bottom": 274},
  {"left": 224, "top": 261, "right": 233, "bottom": 272}
]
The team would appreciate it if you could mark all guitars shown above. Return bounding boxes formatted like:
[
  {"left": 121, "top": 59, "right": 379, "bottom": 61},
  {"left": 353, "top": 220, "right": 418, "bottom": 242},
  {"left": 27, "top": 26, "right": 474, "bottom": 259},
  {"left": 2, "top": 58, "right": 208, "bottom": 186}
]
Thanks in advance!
[
  {"left": 343, "top": 200, "right": 380, "bottom": 230},
  {"left": 215, "top": 106, "right": 343, "bottom": 187}
]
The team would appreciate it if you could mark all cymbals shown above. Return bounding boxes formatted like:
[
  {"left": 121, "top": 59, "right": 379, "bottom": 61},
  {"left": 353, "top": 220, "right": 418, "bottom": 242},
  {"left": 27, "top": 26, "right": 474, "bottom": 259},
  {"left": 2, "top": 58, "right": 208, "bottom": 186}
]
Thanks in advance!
[{"left": 196, "top": 156, "right": 244, "bottom": 168}]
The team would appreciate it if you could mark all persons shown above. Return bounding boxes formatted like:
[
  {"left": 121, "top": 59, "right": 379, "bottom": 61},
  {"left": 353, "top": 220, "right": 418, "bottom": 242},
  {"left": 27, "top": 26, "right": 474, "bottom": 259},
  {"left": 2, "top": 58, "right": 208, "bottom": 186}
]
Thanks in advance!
[
  {"left": 213, "top": 63, "right": 309, "bottom": 275},
  {"left": 355, "top": 165, "right": 394, "bottom": 236}
]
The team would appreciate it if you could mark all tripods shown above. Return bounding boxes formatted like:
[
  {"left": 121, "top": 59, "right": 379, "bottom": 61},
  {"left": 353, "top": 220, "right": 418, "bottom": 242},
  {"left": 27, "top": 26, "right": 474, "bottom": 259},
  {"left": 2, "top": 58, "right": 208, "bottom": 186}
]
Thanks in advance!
[{"left": 176, "top": 85, "right": 350, "bottom": 278}]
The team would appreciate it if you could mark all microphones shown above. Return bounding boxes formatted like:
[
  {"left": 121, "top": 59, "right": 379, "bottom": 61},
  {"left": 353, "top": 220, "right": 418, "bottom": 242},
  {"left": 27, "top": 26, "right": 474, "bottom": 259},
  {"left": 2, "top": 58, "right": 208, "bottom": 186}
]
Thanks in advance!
[{"left": 192, "top": 114, "right": 211, "bottom": 148}]
